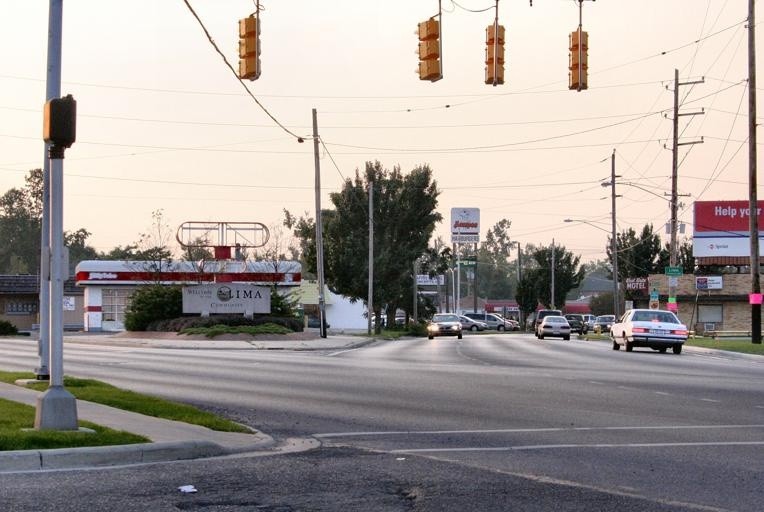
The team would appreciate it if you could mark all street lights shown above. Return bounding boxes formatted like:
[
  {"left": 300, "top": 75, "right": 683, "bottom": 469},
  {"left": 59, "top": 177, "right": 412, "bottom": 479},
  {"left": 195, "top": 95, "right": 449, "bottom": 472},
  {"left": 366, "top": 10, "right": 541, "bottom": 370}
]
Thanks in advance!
[
  {"left": 506, "top": 273, "right": 523, "bottom": 322},
  {"left": 563, "top": 218, "right": 619, "bottom": 316},
  {"left": 599, "top": 182, "right": 681, "bottom": 316}
]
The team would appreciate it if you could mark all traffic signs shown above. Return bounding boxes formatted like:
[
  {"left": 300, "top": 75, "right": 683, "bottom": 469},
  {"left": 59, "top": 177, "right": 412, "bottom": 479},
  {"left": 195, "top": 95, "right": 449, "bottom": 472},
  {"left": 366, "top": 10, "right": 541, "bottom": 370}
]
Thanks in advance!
[{"left": 664, "top": 266, "right": 684, "bottom": 276}]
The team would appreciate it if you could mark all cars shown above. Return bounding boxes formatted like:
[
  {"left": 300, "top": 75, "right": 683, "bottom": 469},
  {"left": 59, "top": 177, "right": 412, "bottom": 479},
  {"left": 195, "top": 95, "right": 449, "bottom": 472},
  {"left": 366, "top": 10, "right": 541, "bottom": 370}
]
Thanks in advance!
[
  {"left": 608, "top": 307, "right": 690, "bottom": 355},
  {"left": 369, "top": 310, "right": 622, "bottom": 341},
  {"left": 290, "top": 308, "right": 330, "bottom": 328}
]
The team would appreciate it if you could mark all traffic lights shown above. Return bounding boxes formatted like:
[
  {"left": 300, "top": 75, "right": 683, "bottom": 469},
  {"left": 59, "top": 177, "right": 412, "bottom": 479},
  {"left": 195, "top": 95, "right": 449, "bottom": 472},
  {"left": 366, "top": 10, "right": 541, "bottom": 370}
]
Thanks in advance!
[{"left": 39, "top": 91, "right": 83, "bottom": 151}]
